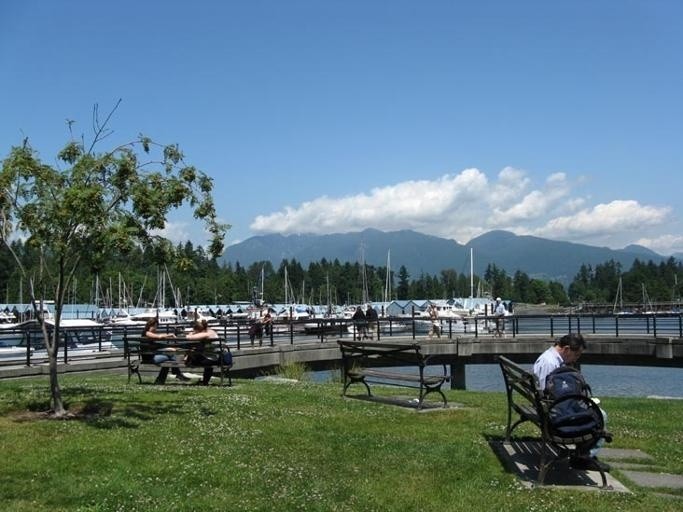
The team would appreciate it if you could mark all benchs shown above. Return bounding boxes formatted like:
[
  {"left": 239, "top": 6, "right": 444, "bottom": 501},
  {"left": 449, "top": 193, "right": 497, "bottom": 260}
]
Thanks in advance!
[
  {"left": 123, "top": 338, "right": 233, "bottom": 386},
  {"left": 500, "top": 355, "right": 613, "bottom": 491},
  {"left": 337, "top": 340, "right": 452, "bottom": 411}
]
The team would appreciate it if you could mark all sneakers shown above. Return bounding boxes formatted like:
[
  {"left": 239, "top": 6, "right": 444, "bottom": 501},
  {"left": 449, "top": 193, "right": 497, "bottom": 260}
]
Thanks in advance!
[
  {"left": 155, "top": 374, "right": 190, "bottom": 385},
  {"left": 569, "top": 455, "right": 610, "bottom": 472}
]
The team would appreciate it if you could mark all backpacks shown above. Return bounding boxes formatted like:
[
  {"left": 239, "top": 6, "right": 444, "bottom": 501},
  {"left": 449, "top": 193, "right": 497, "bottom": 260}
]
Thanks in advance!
[{"left": 544, "top": 363, "right": 602, "bottom": 437}]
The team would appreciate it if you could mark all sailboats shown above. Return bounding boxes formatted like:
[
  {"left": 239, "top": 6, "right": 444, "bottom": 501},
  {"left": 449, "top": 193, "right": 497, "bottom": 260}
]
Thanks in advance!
[{"left": 611, "top": 275, "right": 682, "bottom": 317}]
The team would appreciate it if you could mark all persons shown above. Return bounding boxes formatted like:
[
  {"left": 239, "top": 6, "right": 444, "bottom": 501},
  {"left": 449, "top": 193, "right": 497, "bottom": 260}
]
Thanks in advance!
[
  {"left": 139, "top": 319, "right": 191, "bottom": 384},
  {"left": 258, "top": 313, "right": 272, "bottom": 336},
  {"left": 365, "top": 304, "right": 378, "bottom": 340},
  {"left": 351, "top": 306, "right": 366, "bottom": 341},
  {"left": 532, "top": 333, "right": 611, "bottom": 471},
  {"left": 182, "top": 319, "right": 220, "bottom": 385},
  {"left": 493, "top": 297, "right": 505, "bottom": 337},
  {"left": 428, "top": 303, "right": 441, "bottom": 338},
  {"left": 246, "top": 306, "right": 261, "bottom": 347}
]
